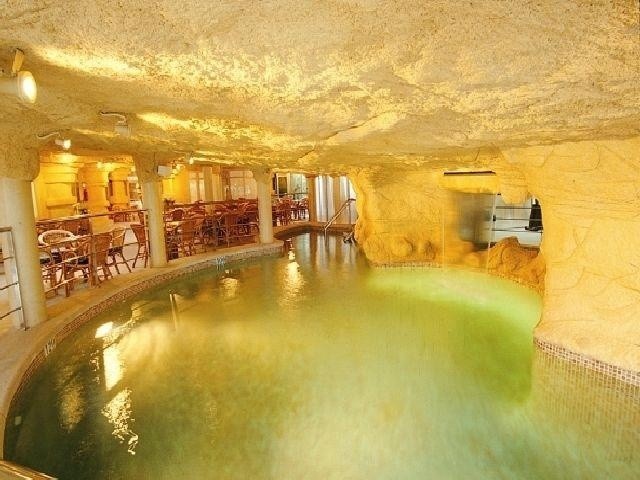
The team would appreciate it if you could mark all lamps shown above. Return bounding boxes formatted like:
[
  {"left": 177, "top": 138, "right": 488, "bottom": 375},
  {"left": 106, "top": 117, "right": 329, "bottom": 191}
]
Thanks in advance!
[{"left": 3, "top": 68, "right": 36, "bottom": 107}]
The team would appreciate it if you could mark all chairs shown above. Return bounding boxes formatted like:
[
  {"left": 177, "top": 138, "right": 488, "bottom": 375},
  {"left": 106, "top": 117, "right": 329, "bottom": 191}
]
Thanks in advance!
[
  {"left": 38, "top": 205, "right": 149, "bottom": 297},
  {"left": 161, "top": 198, "right": 259, "bottom": 262},
  {"left": 271, "top": 195, "right": 308, "bottom": 226}
]
